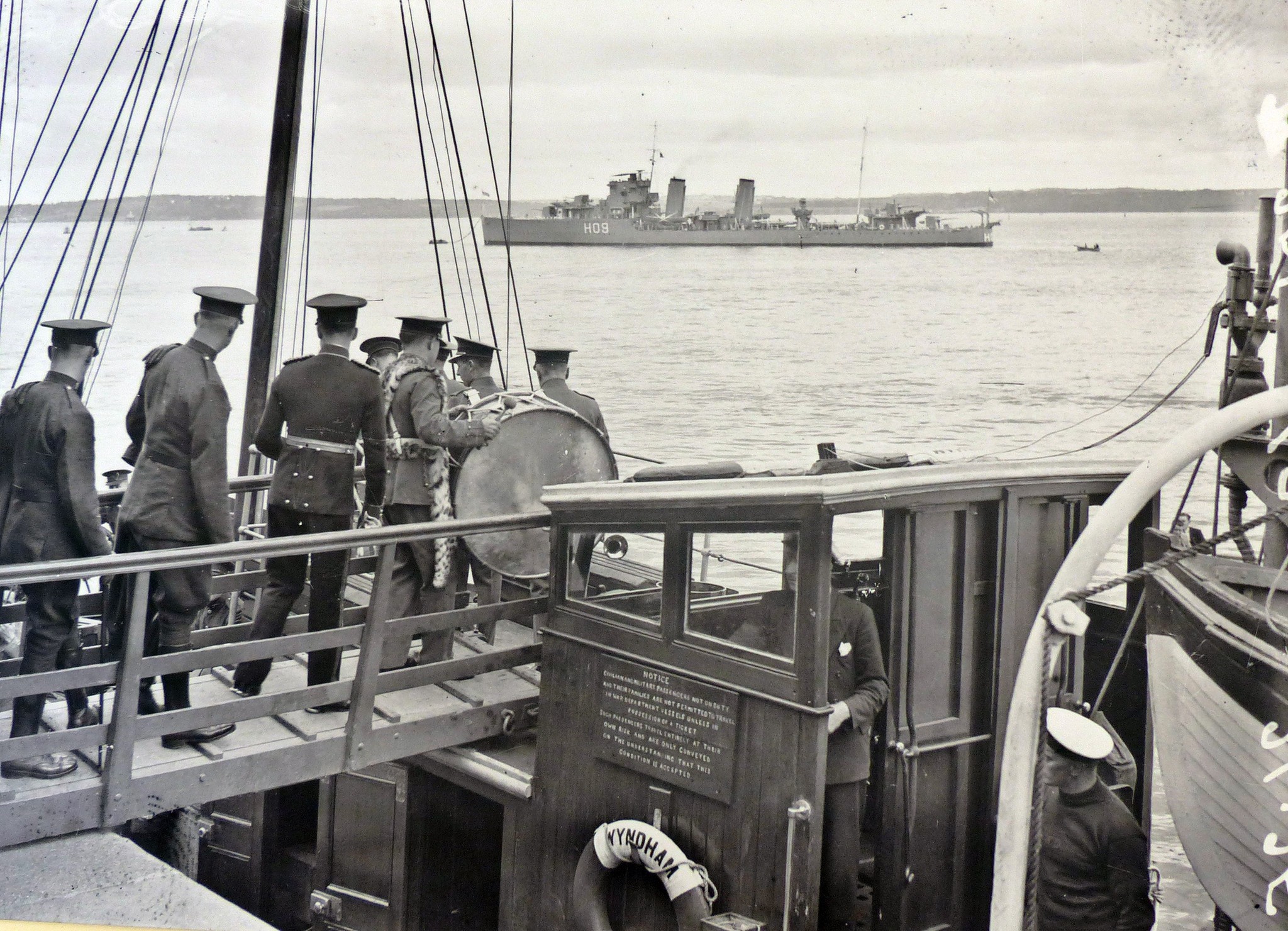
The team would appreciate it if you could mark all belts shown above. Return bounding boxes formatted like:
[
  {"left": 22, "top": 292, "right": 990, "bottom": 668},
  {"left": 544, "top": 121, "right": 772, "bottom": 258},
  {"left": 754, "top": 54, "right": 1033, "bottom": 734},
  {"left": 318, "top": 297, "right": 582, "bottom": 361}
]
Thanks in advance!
[
  {"left": 11, "top": 484, "right": 61, "bottom": 503},
  {"left": 142, "top": 444, "right": 191, "bottom": 471},
  {"left": 384, "top": 438, "right": 442, "bottom": 450},
  {"left": 286, "top": 434, "right": 356, "bottom": 456}
]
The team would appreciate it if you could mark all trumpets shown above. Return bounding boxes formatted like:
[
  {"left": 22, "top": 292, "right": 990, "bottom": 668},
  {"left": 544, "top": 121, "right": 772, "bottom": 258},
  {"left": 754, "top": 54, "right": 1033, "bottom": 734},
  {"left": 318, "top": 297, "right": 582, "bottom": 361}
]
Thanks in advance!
[{"left": 600, "top": 535, "right": 629, "bottom": 560}]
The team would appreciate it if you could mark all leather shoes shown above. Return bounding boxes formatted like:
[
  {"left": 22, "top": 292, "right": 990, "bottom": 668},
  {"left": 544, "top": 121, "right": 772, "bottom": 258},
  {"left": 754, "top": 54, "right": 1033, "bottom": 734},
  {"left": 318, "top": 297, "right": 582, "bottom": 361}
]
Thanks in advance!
[
  {"left": 379, "top": 656, "right": 417, "bottom": 672},
  {"left": 229, "top": 680, "right": 262, "bottom": 696},
  {"left": 305, "top": 700, "right": 350, "bottom": 713}
]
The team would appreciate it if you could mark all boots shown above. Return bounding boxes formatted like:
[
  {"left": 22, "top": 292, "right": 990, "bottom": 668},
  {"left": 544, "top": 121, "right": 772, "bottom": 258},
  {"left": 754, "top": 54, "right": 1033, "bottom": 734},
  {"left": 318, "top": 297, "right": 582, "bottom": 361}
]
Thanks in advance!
[
  {"left": 55, "top": 647, "right": 102, "bottom": 729},
  {"left": 157, "top": 641, "right": 237, "bottom": 749},
  {"left": 122, "top": 651, "right": 164, "bottom": 715},
  {"left": 1, "top": 661, "right": 78, "bottom": 778}
]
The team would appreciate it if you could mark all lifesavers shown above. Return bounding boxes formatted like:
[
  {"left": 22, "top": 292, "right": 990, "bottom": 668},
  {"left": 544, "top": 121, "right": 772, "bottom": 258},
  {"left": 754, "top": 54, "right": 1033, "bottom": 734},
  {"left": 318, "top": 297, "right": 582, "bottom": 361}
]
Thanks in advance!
[{"left": 574, "top": 818, "right": 712, "bottom": 931}]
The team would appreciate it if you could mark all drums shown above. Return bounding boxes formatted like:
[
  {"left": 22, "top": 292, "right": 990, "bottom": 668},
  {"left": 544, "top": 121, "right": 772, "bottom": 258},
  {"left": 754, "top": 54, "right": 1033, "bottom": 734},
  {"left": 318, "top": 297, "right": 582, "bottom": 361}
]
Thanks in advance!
[{"left": 449, "top": 390, "right": 619, "bottom": 579}]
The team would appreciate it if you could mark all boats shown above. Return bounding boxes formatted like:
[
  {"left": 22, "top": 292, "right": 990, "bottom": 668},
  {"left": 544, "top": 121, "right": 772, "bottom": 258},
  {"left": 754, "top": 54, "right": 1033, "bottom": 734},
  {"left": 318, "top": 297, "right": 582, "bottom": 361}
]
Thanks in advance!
[
  {"left": 1075, "top": 244, "right": 1101, "bottom": 252},
  {"left": 482, "top": 146, "right": 999, "bottom": 248}
]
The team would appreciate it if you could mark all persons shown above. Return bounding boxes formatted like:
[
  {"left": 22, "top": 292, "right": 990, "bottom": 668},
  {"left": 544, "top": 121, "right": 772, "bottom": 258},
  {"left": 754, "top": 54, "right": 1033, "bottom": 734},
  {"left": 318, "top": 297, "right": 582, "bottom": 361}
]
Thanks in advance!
[
  {"left": 433, "top": 340, "right": 470, "bottom": 411},
  {"left": 1177, "top": 513, "right": 1212, "bottom": 555},
  {"left": 1034, "top": 705, "right": 1157, "bottom": 931},
  {"left": 379, "top": 314, "right": 502, "bottom": 680},
  {"left": 226, "top": 294, "right": 388, "bottom": 718},
  {"left": 360, "top": 336, "right": 404, "bottom": 374},
  {"left": 448, "top": 335, "right": 507, "bottom": 646},
  {"left": 1172, "top": 519, "right": 1189, "bottom": 549},
  {"left": 728, "top": 532, "right": 891, "bottom": 931},
  {"left": 525, "top": 342, "right": 610, "bottom": 471},
  {"left": 0, "top": 320, "right": 120, "bottom": 780},
  {"left": 111, "top": 285, "right": 262, "bottom": 751}
]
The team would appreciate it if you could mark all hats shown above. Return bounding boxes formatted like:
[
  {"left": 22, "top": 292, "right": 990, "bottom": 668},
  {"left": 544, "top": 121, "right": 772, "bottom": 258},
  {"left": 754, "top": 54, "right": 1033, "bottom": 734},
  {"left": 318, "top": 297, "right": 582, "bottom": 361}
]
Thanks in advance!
[
  {"left": 782, "top": 529, "right": 846, "bottom": 570},
  {"left": 1045, "top": 706, "right": 1115, "bottom": 764},
  {"left": 436, "top": 339, "right": 451, "bottom": 360},
  {"left": 447, "top": 336, "right": 502, "bottom": 363},
  {"left": 526, "top": 347, "right": 578, "bottom": 370},
  {"left": 304, "top": 294, "right": 367, "bottom": 319},
  {"left": 359, "top": 336, "right": 402, "bottom": 364},
  {"left": 40, "top": 319, "right": 113, "bottom": 357},
  {"left": 192, "top": 286, "right": 259, "bottom": 324},
  {"left": 395, "top": 315, "right": 452, "bottom": 347}
]
